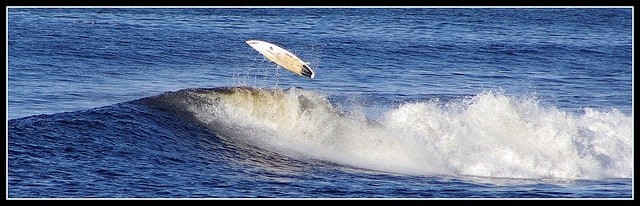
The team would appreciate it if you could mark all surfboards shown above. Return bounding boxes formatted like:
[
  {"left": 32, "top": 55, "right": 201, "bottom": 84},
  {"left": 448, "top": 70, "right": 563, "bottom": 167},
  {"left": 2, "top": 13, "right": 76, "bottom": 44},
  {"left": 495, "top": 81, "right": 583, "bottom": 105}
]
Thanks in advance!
[{"left": 245, "top": 38, "right": 315, "bottom": 80}]
